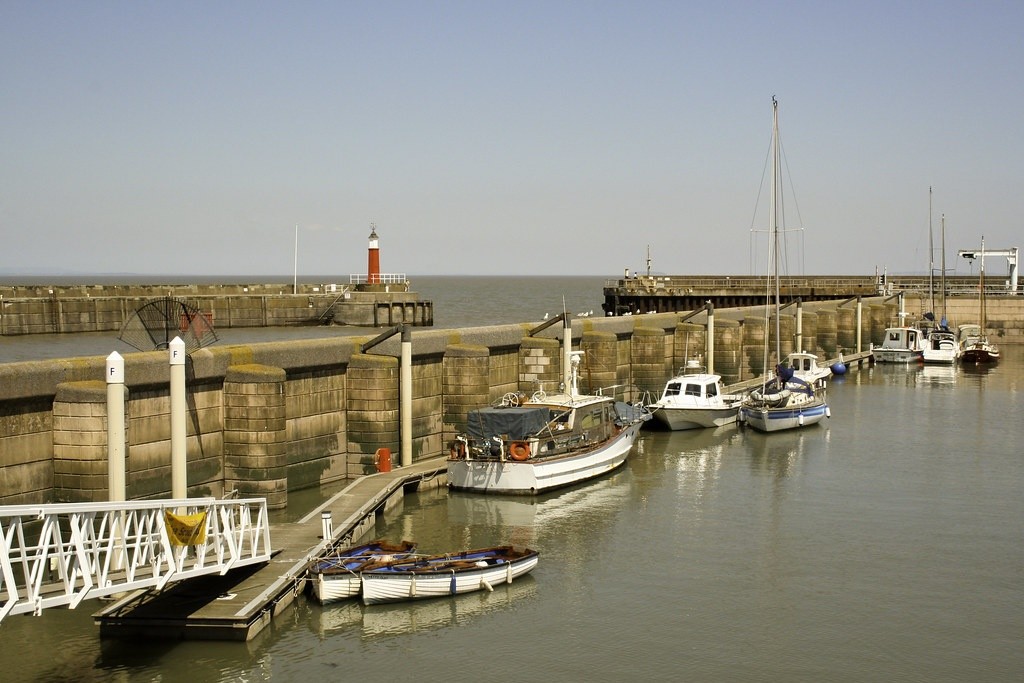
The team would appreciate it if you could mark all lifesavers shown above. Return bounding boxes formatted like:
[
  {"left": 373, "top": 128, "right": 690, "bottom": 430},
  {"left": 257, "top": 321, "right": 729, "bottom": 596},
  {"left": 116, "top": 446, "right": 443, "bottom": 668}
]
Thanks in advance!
[{"left": 510, "top": 442, "right": 531, "bottom": 461}]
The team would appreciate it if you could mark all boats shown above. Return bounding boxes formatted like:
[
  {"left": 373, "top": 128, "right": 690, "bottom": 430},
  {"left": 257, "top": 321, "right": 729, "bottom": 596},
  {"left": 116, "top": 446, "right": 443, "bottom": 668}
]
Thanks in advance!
[
  {"left": 914, "top": 362, "right": 959, "bottom": 387},
  {"left": 633, "top": 300, "right": 749, "bottom": 431},
  {"left": 867, "top": 292, "right": 930, "bottom": 364},
  {"left": 429, "top": 295, "right": 665, "bottom": 498},
  {"left": 313, "top": 537, "right": 418, "bottom": 607},
  {"left": 437, "top": 458, "right": 635, "bottom": 527},
  {"left": 359, "top": 543, "right": 540, "bottom": 607},
  {"left": 361, "top": 573, "right": 538, "bottom": 640},
  {"left": 304, "top": 595, "right": 361, "bottom": 638}
]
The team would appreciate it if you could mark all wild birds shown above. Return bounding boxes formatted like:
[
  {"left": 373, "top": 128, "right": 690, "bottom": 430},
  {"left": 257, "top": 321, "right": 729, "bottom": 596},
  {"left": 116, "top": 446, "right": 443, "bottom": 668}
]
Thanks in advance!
[{"left": 541, "top": 308, "right": 658, "bottom": 322}]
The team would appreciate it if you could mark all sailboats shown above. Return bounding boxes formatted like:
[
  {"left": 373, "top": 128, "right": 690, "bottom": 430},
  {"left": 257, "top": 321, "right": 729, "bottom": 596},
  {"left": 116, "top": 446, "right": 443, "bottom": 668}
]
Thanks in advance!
[
  {"left": 960, "top": 234, "right": 1000, "bottom": 363},
  {"left": 914, "top": 185, "right": 959, "bottom": 365},
  {"left": 729, "top": 94, "right": 838, "bottom": 433}
]
